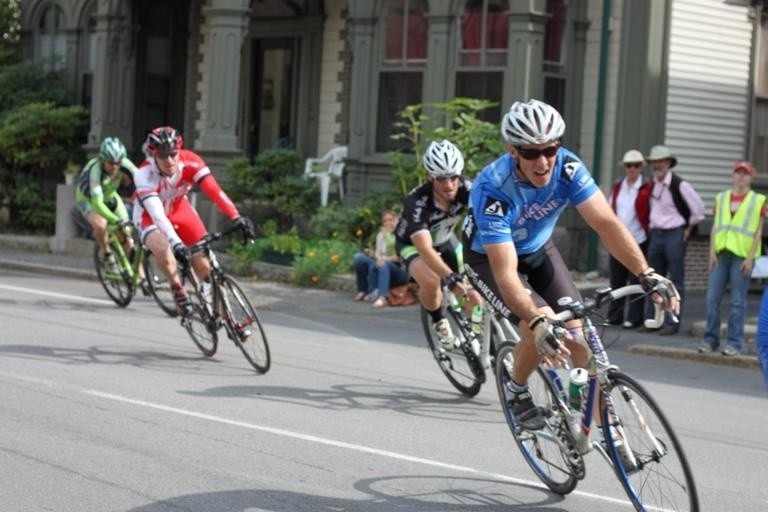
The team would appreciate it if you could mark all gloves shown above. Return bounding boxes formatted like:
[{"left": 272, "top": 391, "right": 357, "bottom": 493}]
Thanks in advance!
[
  {"left": 173, "top": 243, "right": 192, "bottom": 264},
  {"left": 233, "top": 216, "right": 256, "bottom": 239},
  {"left": 117, "top": 220, "right": 132, "bottom": 231}
]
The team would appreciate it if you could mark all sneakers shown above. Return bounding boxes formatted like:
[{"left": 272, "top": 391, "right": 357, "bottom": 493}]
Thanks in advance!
[
  {"left": 142, "top": 285, "right": 161, "bottom": 295},
  {"left": 353, "top": 292, "right": 387, "bottom": 308},
  {"left": 227, "top": 323, "right": 252, "bottom": 340},
  {"left": 431, "top": 318, "right": 461, "bottom": 352},
  {"left": 170, "top": 283, "right": 194, "bottom": 317},
  {"left": 698, "top": 343, "right": 713, "bottom": 353},
  {"left": 601, "top": 439, "right": 638, "bottom": 473},
  {"left": 721, "top": 345, "right": 737, "bottom": 355},
  {"left": 503, "top": 383, "right": 546, "bottom": 431},
  {"left": 103, "top": 254, "right": 120, "bottom": 275}
]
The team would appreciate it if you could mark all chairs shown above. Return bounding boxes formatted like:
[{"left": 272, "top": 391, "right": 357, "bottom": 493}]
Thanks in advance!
[{"left": 303, "top": 146, "right": 348, "bottom": 207}]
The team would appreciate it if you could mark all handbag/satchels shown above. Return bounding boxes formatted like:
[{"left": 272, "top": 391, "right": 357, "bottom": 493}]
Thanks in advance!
[{"left": 388, "top": 283, "right": 417, "bottom": 306}]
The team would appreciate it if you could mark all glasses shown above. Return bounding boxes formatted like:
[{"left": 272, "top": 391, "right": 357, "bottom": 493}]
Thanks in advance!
[
  {"left": 157, "top": 150, "right": 178, "bottom": 160},
  {"left": 625, "top": 163, "right": 639, "bottom": 168},
  {"left": 435, "top": 175, "right": 459, "bottom": 182},
  {"left": 517, "top": 145, "right": 559, "bottom": 160}
]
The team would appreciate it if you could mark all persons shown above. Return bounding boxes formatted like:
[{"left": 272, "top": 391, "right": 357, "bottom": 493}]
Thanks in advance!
[
  {"left": 698, "top": 162, "right": 768, "bottom": 356},
  {"left": 133, "top": 127, "right": 254, "bottom": 342},
  {"left": 461, "top": 98, "right": 681, "bottom": 475},
  {"left": 603, "top": 149, "right": 657, "bottom": 328},
  {"left": 76, "top": 136, "right": 138, "bottom": 276},
  {"left": 392, "top": 139, "right": 484, "bottom": 351},
  {"left": 117, "top": 172, "right": 137, "bottom": 222},
  {"left": 355, "top": 210, "right": 412, "bottom": 308},
  {"left": 637, "top": 145, "right": 705, "bottom": 336}
]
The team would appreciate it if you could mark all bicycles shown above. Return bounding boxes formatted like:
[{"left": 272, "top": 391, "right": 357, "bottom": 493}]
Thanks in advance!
[
  {"left": 493, "top": 282, "right": 699, "bottom": 511},
  {"left": 419, "top": 259, "right": 520, "bottom": 395},
  {"left": 172, "top": 222, "right": 274, "bottom": 374},
  {"left": 93, "top": 224, "right": 186, "bottom": 317}
]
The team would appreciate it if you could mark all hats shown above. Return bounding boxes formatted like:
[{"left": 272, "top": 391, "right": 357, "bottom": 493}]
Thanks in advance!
[
  {"left": 645, "top": 145, "right": 677, "bottom": 168},
  {"left": 619, "top": 149, "right": 645, "bottom": 168},
  {"left": 733, "top": 162, "right": 752, "bottom": 176}
]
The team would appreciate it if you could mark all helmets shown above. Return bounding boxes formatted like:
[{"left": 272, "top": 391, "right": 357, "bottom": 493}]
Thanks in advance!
[
  {"left": 501, "top": 98, "right": 566, "bottom": 146},
  {"left": 146, "top": 126, "right": 183, "bottom": 153},
  {"left": 100, "top": 136, "right": 128, "bottom": 163},
  {"left": 422, "top": 140, "right": 465, "bottom": 179}
]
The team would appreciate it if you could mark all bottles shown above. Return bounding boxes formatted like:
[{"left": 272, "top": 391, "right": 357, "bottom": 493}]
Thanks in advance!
[
  {"left": 472, "top": 304, "right": 483, "bottom": 334},
  {"left": 568, "top": 368, "right": 589, "bottom": 412},
  {"left": 202, "top": 275, "right": 215, "bottom": 305}
]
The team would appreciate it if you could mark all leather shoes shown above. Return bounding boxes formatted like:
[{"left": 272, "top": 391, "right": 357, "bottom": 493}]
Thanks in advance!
[{"left": 603, "top": 319, "right": 679, "bottom": 336}]
